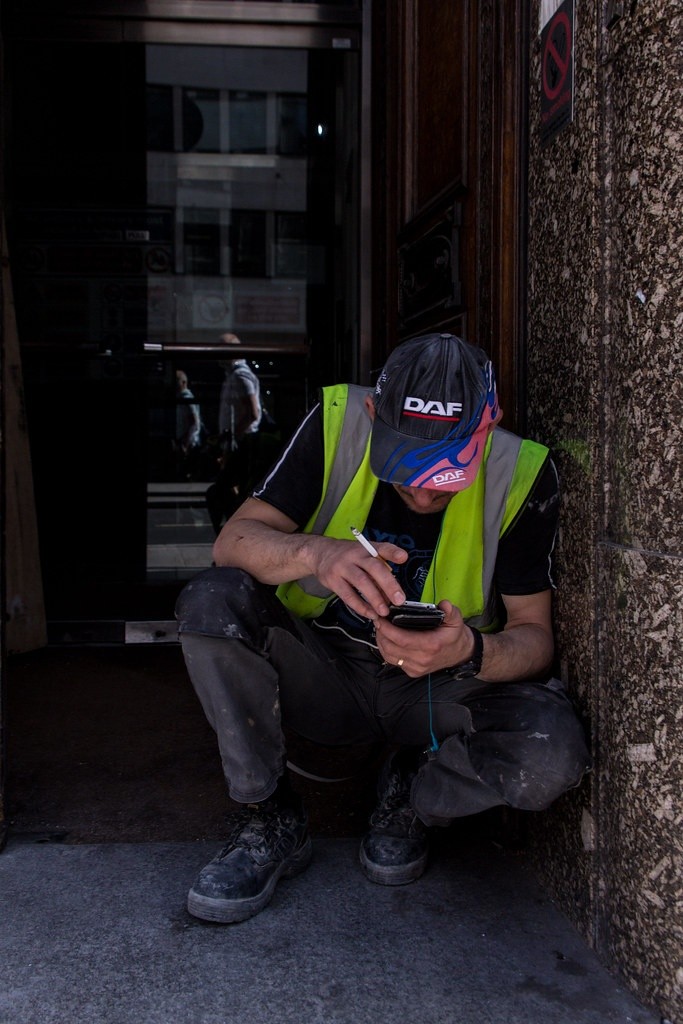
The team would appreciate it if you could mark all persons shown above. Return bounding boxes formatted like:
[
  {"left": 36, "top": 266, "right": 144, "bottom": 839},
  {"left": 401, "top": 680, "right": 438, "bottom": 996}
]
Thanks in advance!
[
  {"left": 169, "top": 371, "right": 206, "bottom": 467},
  {"left": 178, "top": 334, "right": 598, "bottom": 925},
  {"left": 212, "top": 335, "right": 269, "bottom": 530}
]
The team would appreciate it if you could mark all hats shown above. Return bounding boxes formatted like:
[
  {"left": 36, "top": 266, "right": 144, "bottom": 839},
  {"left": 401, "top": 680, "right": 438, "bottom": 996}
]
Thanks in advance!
[{"left": 370, "top": 333, "right": 503, "bottom": 492}]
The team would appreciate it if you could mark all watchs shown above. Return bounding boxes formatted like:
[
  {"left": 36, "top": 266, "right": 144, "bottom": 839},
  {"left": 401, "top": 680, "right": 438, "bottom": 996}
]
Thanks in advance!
[{"left": 441, "top": 624, "right": 483, "bottom": 682}]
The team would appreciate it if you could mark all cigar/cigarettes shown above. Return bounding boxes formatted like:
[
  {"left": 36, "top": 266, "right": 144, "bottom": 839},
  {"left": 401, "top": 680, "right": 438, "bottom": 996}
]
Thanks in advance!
[{"left": 351, "top": 526, "right": 392, "bottom": 573}]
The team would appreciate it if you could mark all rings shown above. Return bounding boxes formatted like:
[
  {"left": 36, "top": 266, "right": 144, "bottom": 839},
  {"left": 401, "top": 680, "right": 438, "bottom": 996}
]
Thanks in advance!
[{"left": 397, "top": 657, "right": 403, "bottom": 667}]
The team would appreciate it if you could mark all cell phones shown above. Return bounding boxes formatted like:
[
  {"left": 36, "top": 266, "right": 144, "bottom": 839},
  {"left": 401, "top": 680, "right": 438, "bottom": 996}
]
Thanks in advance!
[{"left": 387, "top": 600, "right": 445, "bottom": 630}]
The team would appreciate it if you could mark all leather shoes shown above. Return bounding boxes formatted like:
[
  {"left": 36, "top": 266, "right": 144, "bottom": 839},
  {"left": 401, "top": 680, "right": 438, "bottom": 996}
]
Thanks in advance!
[
  {"left": 360, "top": 745, "right": 428, "bottom": 885},
  {"left": 187, "top": 797, "right": 312, "bottom": 922}
]
